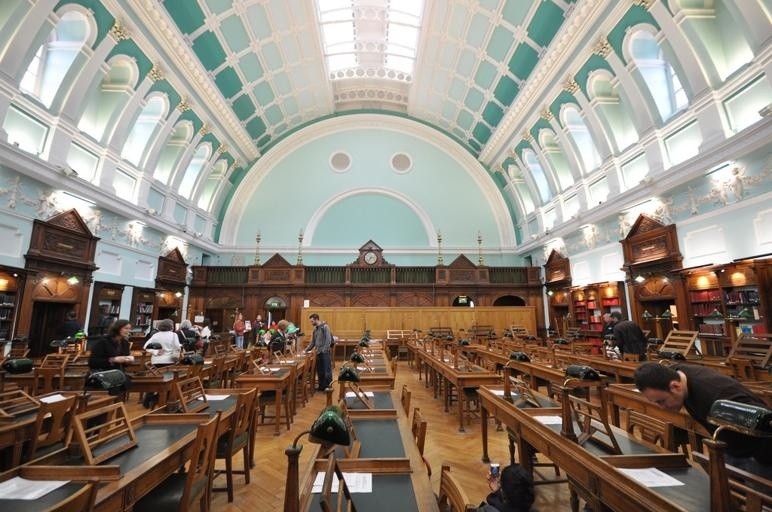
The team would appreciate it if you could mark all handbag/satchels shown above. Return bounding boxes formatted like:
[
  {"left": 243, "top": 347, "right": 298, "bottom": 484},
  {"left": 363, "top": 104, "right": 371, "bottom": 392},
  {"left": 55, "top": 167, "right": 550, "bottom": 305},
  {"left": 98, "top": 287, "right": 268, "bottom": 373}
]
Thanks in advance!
[{"left": 320, "top": 323, "right": 334, "bottom": 348}]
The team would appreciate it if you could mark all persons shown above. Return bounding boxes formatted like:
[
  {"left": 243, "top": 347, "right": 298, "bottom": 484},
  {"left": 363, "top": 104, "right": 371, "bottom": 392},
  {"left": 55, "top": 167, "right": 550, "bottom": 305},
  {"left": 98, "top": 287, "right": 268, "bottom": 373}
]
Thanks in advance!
[
  {"left": 301, "top": 314, "right": 332, "bottom": 392},
  {"left": 633, "top": 362, "right": 771, "bottom": 468},
  {"left": 60, "top": 311, "right": 214, "bottom": 438},
  {"left": 599, "top": 311, "right": 648, "bottom": 382},
  {"left": 232, "top": 311, "right": 293, "bottom": 356},
  {"left": 476, "top": 463, "right": 538, "bottom": 512}
]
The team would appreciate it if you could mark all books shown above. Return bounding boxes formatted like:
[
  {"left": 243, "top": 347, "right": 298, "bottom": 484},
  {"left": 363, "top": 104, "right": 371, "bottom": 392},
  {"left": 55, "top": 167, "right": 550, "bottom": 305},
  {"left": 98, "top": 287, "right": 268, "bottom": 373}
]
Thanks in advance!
[
  {"left": 98, "top": 303, "right": 120, "bottom": 314},
  {"left": 134, "top": 303, "right": 153, "bottom": 327}
]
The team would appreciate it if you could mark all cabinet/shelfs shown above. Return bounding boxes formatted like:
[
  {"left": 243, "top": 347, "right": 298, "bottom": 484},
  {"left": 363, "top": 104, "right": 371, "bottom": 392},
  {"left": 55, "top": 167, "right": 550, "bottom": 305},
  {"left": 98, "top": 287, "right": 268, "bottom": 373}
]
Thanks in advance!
[
  {"left": 89, "top": 281, "right": 126, "bottom": 330},
  {"left": 0, "top": 264, "right": 39, "bottom": 342},
  {"left": 129, "top": 285, "right": 166, "bottom": 328},
  {"left": 572, "top": 283, "right": 628, "bottom": 331},
  {"left": 682, "top": 258, "right": 772, "bottom": 335}
]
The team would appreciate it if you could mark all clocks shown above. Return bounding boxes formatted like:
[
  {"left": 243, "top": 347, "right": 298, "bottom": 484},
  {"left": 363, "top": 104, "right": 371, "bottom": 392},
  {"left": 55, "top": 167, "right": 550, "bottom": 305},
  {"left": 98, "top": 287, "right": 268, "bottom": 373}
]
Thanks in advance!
[{"left": 364, "top": 252, "right": 378, "bottom": 265}]
